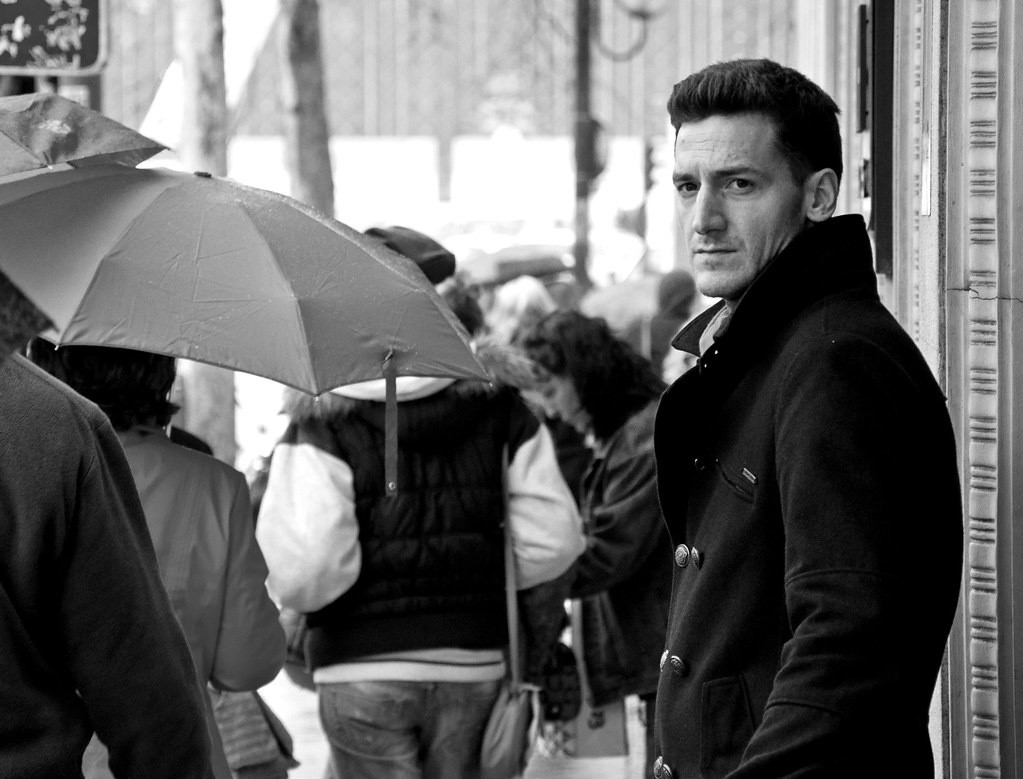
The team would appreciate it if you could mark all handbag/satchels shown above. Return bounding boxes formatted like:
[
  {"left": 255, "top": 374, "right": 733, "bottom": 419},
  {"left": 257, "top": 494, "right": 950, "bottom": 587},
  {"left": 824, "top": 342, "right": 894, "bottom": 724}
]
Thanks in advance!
[
  {"left": 480, "top": 680, "right": 532, "bottom": 779},
  {"left": 537, "top": 672, "right": 628, "bottom": 758}
]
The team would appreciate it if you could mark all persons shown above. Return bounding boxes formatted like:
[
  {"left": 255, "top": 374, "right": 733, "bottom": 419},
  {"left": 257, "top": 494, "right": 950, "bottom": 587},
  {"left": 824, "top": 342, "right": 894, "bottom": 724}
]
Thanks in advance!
[
  {"left": 28, "top": 334, "right": 285, "bottom": 779},
  {"left": 0, "top": 269, "right": 215, "bottom": 779},
  {"left": 256, "top": 224, "right": 706, "bottom": 779},
  {"left": 653, "top": 58, "right": 965, "bottom": 779}
]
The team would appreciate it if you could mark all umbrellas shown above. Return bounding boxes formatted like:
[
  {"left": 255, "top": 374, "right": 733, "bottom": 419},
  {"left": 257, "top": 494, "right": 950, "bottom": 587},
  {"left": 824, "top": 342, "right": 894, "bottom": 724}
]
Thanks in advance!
[
  {"left": 0, "top": 93, "right": 170, "bottom": 211},
  {"left": 1, "top": 170, "right": 492, "bottom": 495}
]
